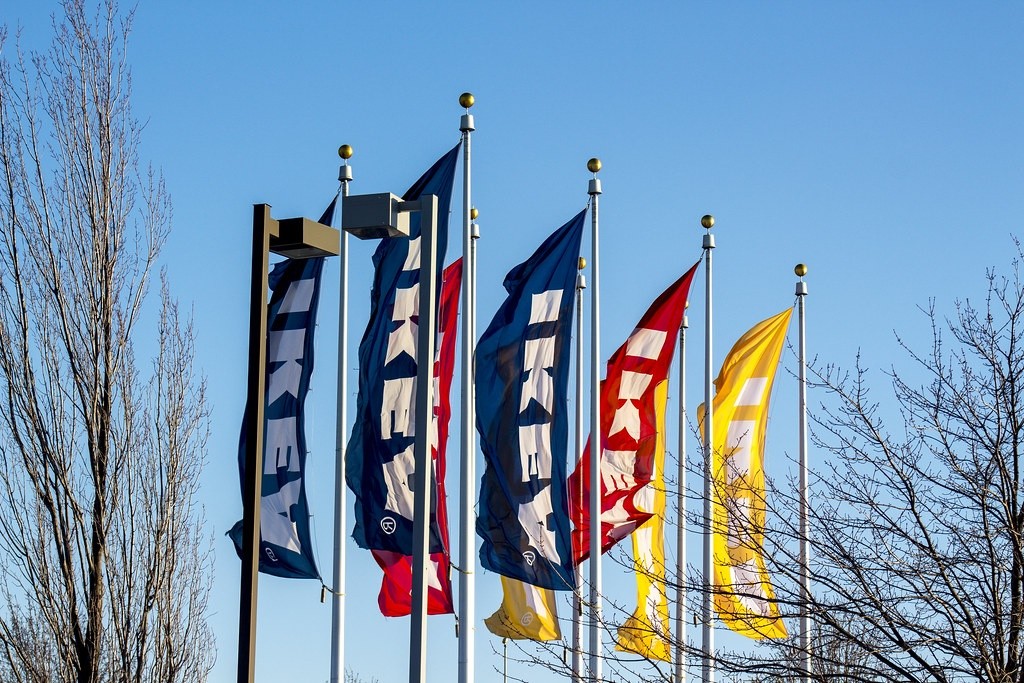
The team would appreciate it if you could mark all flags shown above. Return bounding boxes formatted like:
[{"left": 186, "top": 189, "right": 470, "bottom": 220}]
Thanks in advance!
[
  {"left": 475, "top": 208, "right": 588, "bottom": 640},
  {"left": 344, "top": 141, "right": 463, "bottom": 556},
  {"left": 614, "top": 378, "right": 672, "bottom": 662},
  {"left": 568, "top": 261, "right": 698, "bottom": 567},
  {"left": 362, "top": 256, "right": 464, "bottom": 617},
  {"left": 228, "top": 193, "right": 340, "bottom": 578},
  {"left": 696, "top": 306, "right": 795, "bottom": 640}
]
